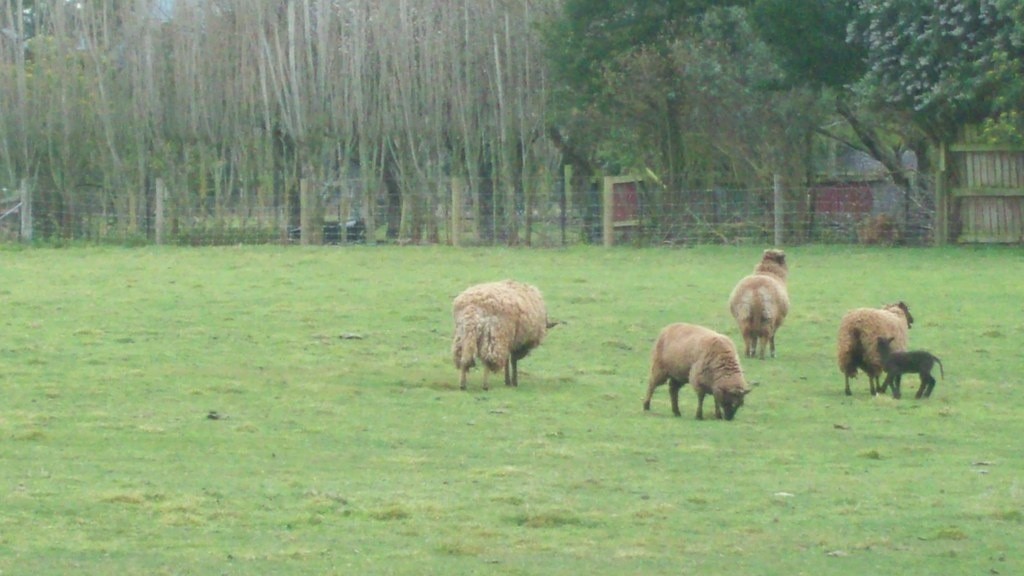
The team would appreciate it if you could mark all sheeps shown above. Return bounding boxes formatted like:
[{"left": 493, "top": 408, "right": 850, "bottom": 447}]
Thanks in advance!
[
  {"left": 837, "top": 301, "right": 915, "bottom": 397},
  {"left": 875, "top": 335, "right": 945, "bottom": 400},
  {"left": 643, "top": 322, "right": 752, "bottom": 421},
  {"left": 730, "top": 248, "right": 790, "bottom": 362},
  {"left": 453, "top": 278, "right": 558, "bottom": 391}
]
